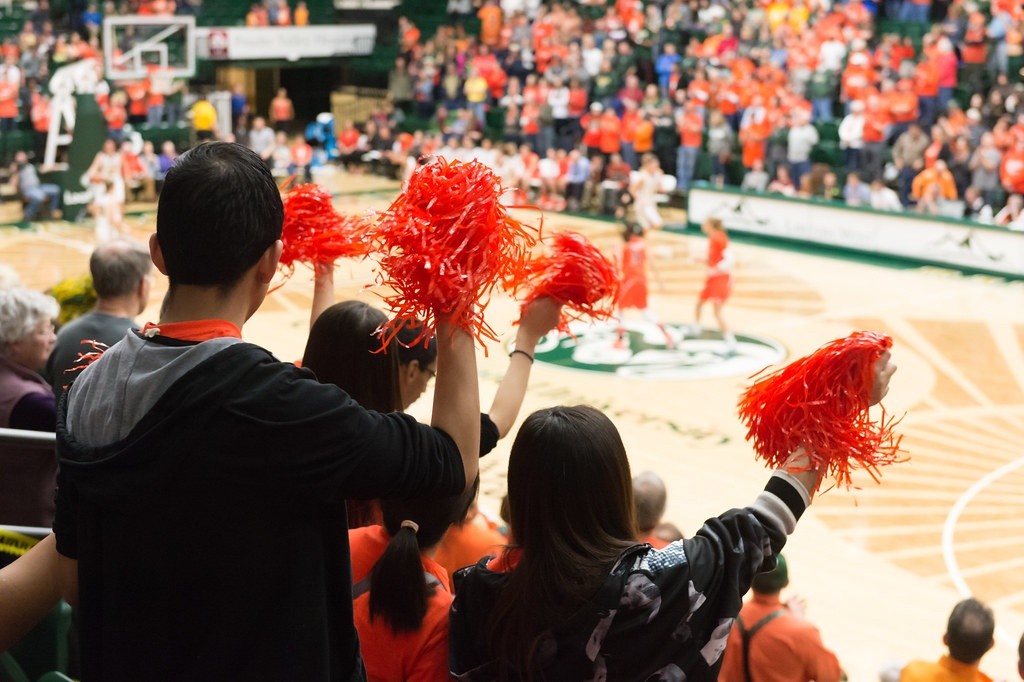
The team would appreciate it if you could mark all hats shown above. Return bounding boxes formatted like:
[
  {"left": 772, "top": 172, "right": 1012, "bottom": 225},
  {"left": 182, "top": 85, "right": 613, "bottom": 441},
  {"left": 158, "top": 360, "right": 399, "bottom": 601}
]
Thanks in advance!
[{"left": 750, "top": 553, "right": 787, "bottom": 587}]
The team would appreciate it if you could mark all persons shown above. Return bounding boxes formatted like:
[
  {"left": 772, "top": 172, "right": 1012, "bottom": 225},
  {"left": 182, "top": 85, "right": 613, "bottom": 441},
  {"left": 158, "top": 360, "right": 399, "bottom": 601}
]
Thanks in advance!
[
  {"left": 387, "top": 314, "right": 437, "bottom": 412},
  {"left": 718, "top": 552, "right": 848, "bottom": 681},
  {"left": 631, "top": 465, "right": 672, "bottom": 549},
  {"left": 683, "top": 217, "right": 737, "bottom": 343},
  {"left": 879, "top": 599, "right": 996, "bottom": 682},
  {"left": 454, "top": 344, "right": 897, "bottom": 682},
  {"left": 52, "top": 142, "right": 481, "bottom": 681},
  {"left": 614, "top": 220, "right": 683, "bottom": 349},
  {"left": 0, "top": 286, "right": 60, "bottom": 433},
  {"left": 301, "top": 297, "right": 560, "bottom": 457},
  {"left": 348, "top": 470, "right": 479, "bottom": 682},
  {"left": 45, "top": 240, "right": 154, "bottom": 405},
  {"left": 0, "top": 0, "right": 1023, "bottom": 248}
]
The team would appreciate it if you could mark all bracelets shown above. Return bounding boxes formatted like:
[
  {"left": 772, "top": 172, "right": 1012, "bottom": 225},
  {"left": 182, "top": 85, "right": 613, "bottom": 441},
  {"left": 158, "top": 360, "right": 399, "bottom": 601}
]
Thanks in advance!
[{"left": 510, "top": 350, "right": 533, "bottom": 364}]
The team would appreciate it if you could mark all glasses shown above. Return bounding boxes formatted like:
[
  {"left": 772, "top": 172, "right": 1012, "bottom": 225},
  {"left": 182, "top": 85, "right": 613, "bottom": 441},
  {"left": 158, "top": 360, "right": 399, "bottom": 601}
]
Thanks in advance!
[{"left": 419, "top": 364, "right": 437, "bottom": 386}]
